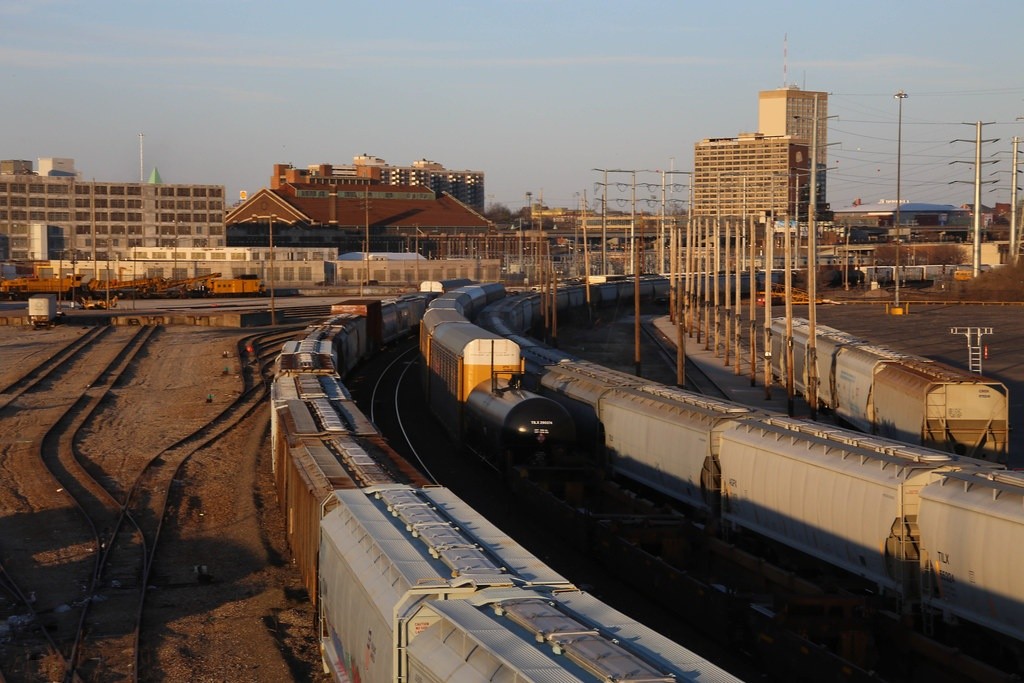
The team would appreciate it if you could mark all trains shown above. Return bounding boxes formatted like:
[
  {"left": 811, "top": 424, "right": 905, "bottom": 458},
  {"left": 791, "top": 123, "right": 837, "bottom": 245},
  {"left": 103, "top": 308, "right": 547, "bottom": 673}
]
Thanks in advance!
[
  {"left": 269, "top": 278, "right": 742, "bottom": 683},
  {"left": 479, "top": 267, "right": 1024, "bottom": 650},
  {"left": 416, "top": 280, "right": 580, "bottom": 486},
  {"left": 764, "top": 314, "right": 1010, "bottom": 464}
]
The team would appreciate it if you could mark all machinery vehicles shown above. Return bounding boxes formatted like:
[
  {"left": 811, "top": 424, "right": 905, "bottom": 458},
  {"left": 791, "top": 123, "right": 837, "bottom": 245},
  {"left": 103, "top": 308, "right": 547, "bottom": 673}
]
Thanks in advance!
[
  {"left": 202, "top": 277, "right": 267, "bottom": 298},
  {"left": 156, "top": 272, "right": 222, "bottom": 297},
  {"left": 0, "top": 276, "right": 86, "bottom": 301},
  {"left": 84, "top": 276, "right": 160, "bottom": 300}
]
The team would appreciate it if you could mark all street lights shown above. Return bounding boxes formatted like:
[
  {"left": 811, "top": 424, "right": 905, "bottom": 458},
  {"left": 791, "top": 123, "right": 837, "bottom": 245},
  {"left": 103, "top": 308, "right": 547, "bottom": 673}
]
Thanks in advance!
[{"left": 892, "top": 90, "right": 908, "bottom": 309}]
